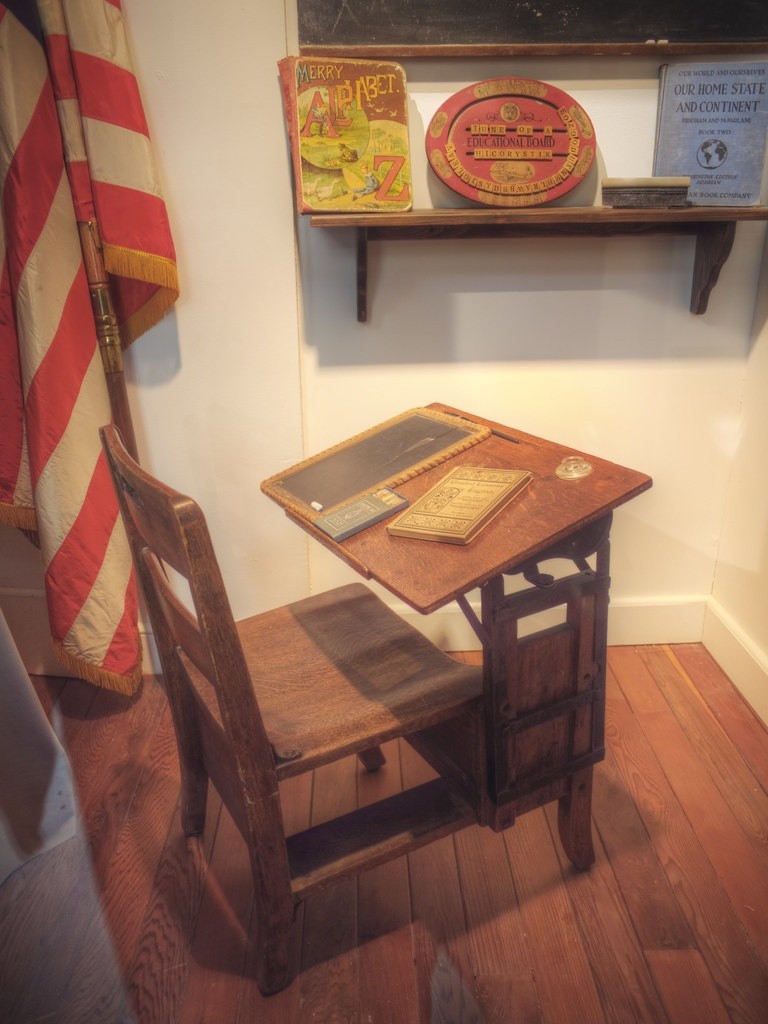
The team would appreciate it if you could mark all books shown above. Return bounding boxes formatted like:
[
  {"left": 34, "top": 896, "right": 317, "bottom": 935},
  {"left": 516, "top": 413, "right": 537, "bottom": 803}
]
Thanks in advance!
[
  {"left": 386, "top": 466, "right": 534, "bottom": 546},
  {"left": 280, "top": 56, "right": 414, "bottom": 216}
]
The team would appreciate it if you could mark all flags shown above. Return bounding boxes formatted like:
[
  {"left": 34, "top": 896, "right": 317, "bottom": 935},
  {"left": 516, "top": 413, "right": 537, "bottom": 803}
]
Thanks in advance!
[{"left": 2, "top": 0, "right": 182, "bottom": 698}]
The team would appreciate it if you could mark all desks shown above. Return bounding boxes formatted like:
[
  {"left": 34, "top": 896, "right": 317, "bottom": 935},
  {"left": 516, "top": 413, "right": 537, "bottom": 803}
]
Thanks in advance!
[{"left": 256, "top": 402, "right": 654, "bottom": 873}]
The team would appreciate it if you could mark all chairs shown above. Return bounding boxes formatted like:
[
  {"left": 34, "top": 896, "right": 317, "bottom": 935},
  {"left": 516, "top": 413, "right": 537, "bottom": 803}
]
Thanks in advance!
[{"left": 98, "top": 422, "right": 493, "bottom": 995}]
